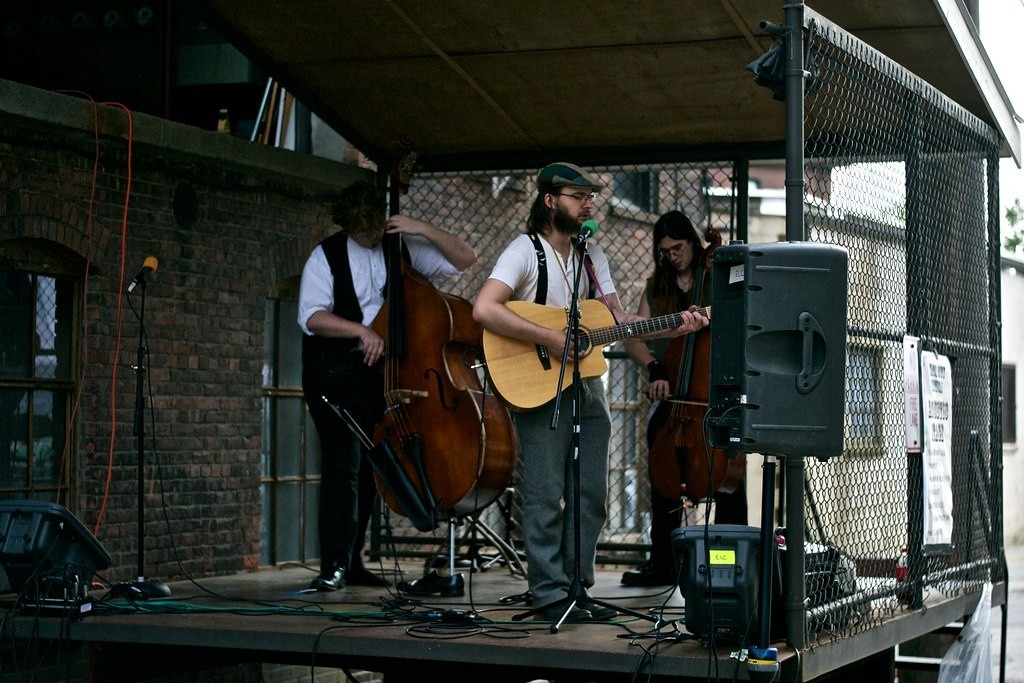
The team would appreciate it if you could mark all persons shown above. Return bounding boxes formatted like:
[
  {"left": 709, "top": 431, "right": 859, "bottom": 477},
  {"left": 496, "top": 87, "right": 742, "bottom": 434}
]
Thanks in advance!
[
  {"left": 298, "top": 179, "right": 475, "bottom": 591},
  {"left": 473, "top": 163, "right": 708, "bottom": 622},
  {"left": 621, "top": 210, "right": 744, "bottom": 587}
]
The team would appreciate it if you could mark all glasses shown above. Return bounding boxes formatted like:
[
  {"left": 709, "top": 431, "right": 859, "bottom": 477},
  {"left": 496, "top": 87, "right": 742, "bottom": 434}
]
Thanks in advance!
[
  {"left": 660, "top": 243, "right": 688, "bottom": 258},
  {"left": 561, "top": 194, "right": 597, "bottom": 204}
]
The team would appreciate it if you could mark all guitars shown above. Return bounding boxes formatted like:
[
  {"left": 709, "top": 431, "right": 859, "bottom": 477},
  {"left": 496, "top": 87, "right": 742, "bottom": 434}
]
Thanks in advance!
[{"left": 481, "top": 298, "right": 713, "bottom": 416}]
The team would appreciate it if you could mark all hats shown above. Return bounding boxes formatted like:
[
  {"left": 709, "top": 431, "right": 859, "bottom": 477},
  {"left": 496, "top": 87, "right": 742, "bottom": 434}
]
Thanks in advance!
[
  {"left": 537, "top": 162, "right": 606, "bottom": 193},
  {"left": 331, "top": 180, "right": 384, "bottom": 225}
]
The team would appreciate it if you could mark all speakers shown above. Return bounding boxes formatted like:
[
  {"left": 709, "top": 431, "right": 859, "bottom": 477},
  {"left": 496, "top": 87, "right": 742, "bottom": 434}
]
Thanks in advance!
[
  {"left": 0, "top": 499, "right": 111, "bottom": 606},
  {"left": 670, "top": 524, "right": 783, "bottom": 649},
  {"left": 709, "top": 240, "right": 848, "bottom": 462}
]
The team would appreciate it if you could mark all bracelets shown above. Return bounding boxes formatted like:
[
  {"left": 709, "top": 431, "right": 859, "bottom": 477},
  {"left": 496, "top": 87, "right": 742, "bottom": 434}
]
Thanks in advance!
[{"left": 647, "top": 360, "right": 660, "bottom": 369}]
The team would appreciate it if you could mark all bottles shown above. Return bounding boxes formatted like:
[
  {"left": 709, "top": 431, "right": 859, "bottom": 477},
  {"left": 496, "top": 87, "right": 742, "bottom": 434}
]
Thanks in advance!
[{"left": 895, "top": 548, "right": 909, "bottom": 605}]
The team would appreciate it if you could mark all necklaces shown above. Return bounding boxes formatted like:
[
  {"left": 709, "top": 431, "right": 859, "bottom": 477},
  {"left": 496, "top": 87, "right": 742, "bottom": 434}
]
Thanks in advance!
[
  {"left": 544, "top": 236, "right": 577, "bottom": 299},
  {"left": 678, "top": 275, "right": 692, "bottom": 293}
]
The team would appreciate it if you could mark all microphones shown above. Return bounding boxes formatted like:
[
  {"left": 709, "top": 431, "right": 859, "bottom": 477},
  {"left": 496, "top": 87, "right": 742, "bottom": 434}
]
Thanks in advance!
[
  {"left": 128, "top": 257, "right": 158, "bottom": 292},
  {"left": 577, "top": 219, "right": 598, "bottom": 244}
]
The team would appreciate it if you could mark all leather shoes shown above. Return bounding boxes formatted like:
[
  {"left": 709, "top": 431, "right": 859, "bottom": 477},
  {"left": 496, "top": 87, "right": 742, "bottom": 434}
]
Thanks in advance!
[
  {"left": 534, "top": 599, "right": 620, "bottom": 622},
  {"left": 396, "top": 569, "right": 466, "bottom": 599},
  {"left": 309, "top": 565, "right": 346, "bottom": 591},
  {"left": 621, "top": 570, "right": 677, "bottom": 587}
]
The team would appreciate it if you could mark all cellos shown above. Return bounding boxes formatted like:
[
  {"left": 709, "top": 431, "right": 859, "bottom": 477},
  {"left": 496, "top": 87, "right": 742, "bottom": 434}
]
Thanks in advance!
[
  {"left": 645, "top": 226, "right": 749, "bottom": 503},
  {"left": 356, "top": 119, "right": 519, "bottom": 576}
]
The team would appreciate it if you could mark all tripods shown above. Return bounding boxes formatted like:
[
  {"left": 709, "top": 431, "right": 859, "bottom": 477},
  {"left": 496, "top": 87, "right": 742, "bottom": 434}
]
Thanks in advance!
[{"left": 510, "top": 241, "right": 668, "bottom": 635}]
